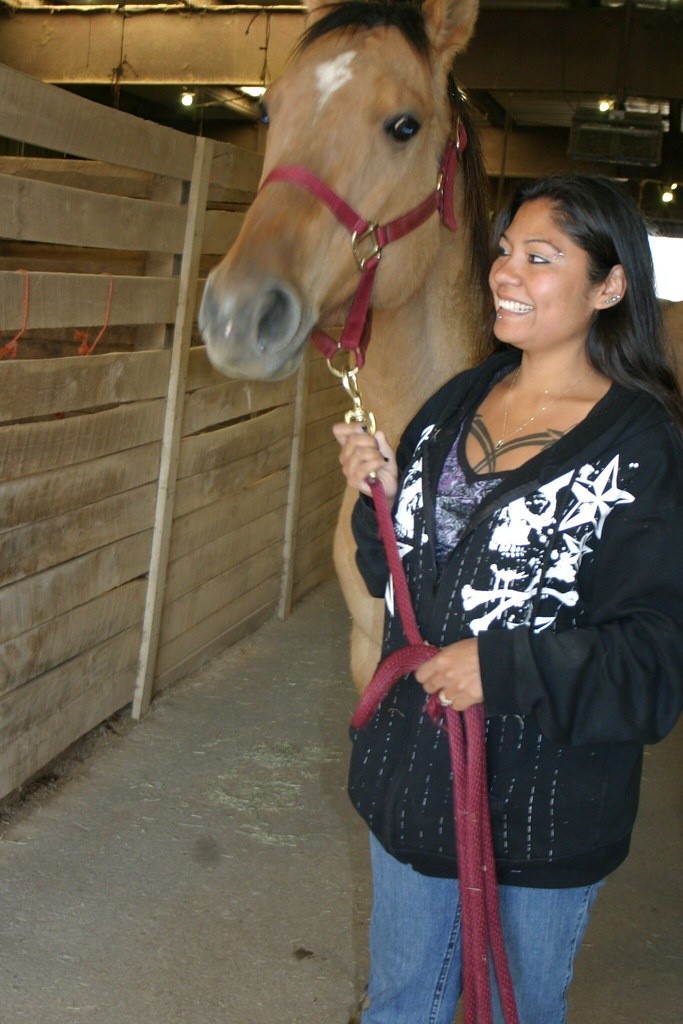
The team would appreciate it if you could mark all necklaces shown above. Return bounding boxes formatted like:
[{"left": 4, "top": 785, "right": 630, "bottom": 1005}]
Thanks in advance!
[{"left": 494, "top": 363, "right": 597, "bottom": 450}]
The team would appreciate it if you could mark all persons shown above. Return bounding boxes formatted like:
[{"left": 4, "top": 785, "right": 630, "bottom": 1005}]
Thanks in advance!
[{"left": 332, "top": 170, "right": 683, "bottom": 1024}]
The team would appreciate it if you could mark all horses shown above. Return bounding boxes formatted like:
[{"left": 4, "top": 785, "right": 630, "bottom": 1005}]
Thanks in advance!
[{"left": 198, "top": 0, "right": 683, "bottom": 698}]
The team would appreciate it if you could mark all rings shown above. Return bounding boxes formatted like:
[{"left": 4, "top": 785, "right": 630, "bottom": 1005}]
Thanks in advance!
[{"left": 437, "top": 690, "right": 453, "bottom": 707}]
